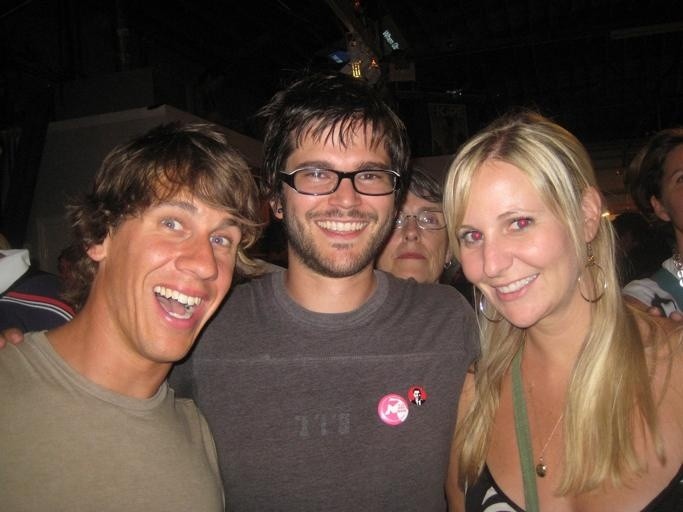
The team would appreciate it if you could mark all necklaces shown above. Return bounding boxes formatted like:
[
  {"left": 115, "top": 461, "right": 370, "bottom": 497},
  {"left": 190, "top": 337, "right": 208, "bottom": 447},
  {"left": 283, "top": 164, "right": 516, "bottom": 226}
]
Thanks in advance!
[
  {"left": 671, "top": 248, "right": 683, "bottom": 287},
  {"left": 522, "top": 359, "right": 565, "bottom": 477}
]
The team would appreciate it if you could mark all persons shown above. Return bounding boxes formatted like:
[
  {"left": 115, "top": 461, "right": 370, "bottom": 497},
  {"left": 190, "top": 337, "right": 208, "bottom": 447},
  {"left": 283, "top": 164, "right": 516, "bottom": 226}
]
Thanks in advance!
[
  {"left": 376, "top": 164, "right": 457, "bottom": 287},
  {"left": 611, "top": 209, "right": 673, "bottom": 285},
  {"left": 0, "top": 234, "right": 84, "bottom": 338},
  {"left": 1, "top": 119, "right": 274, "bottom": 511},
  {"left": 436, "top": 106, "right": 683, "bottom": 511},
  {"left": 615, "top": 126, "right": 682, "bottom": 325},
  {"left": 0, "top": 77, "right": 483, "bottom": 512}
]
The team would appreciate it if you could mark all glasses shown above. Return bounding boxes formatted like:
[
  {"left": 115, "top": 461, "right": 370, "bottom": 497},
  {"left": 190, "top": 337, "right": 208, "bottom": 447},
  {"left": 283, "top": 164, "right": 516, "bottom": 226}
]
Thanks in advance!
[
  {"left": 278, "top": 168, "right": 400, "bottom": 196},
  {"left": 394, "top": 211, "right": 446, "bottom": 230}
]
